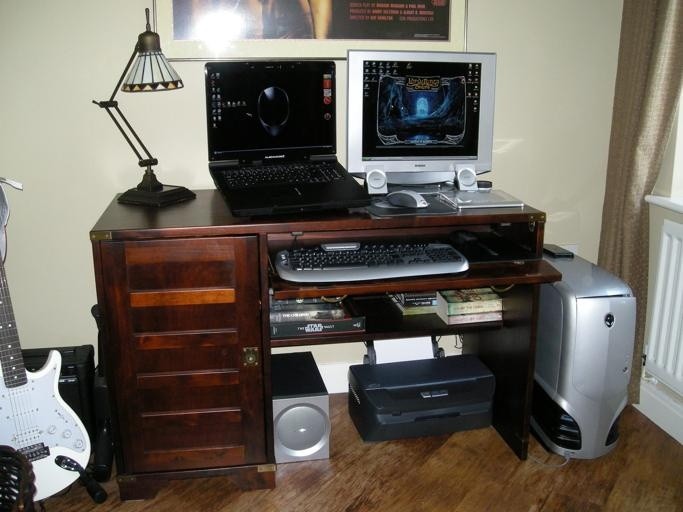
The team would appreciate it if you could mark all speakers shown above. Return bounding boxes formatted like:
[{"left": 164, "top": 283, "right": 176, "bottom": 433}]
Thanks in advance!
[{"left": 272, "top": 351, "right": 331, "bottom": 464}]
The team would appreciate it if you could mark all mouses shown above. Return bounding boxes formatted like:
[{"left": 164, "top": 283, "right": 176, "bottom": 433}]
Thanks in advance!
[{"left": 386, "top": 190, "right": 428, "bottom": 208}]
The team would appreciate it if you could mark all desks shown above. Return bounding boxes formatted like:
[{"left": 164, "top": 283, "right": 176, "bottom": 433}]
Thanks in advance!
[{"left": 89, "top": 185, "right": 564, "bottom": 502}]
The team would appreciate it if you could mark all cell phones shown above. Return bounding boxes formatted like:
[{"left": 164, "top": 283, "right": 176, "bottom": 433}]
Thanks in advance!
[{"left": 543, "top": 244, "right": 573, "bottom": 258}]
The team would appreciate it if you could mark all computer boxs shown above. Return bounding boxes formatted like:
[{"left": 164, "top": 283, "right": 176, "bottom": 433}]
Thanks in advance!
[{"left": 529, "top": 252, "right": 636, "bottom": 460}]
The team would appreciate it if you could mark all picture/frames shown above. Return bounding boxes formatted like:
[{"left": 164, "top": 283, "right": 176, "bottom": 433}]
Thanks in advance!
[{"left": 152, "top": 0, "right": 468, "bottom": 62}]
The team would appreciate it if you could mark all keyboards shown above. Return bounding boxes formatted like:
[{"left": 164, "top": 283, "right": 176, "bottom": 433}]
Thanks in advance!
[{"left": 275, "top": 242, "right": 469, "bottom": 283}]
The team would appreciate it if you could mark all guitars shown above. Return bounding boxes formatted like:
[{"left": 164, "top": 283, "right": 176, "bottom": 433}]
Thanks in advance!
[{"left": 0, "top": 177, "right": 91, "bottom": 502}]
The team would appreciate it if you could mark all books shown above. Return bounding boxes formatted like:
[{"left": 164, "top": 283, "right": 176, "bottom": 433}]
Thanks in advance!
[
  {"left": 269, "top": 294, "right": 344, "bottom": 324},
  {"left": 386, "top": 287, "right": 503, "bottom": 325}
]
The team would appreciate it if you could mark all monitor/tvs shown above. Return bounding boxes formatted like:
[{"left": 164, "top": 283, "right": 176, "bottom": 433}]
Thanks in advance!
[{"left": 348, "top": 50, "right": 497, "bottom": 185}]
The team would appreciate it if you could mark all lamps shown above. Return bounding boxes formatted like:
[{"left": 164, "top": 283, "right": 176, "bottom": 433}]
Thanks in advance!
[{"left": 92, "top": 6, "right": 197, "bottom": 210}]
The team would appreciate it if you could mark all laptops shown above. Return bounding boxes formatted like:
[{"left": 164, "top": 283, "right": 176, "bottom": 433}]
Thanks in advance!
[{"left": 205, "top": 61, "right": 371, "bottom": 218}]
